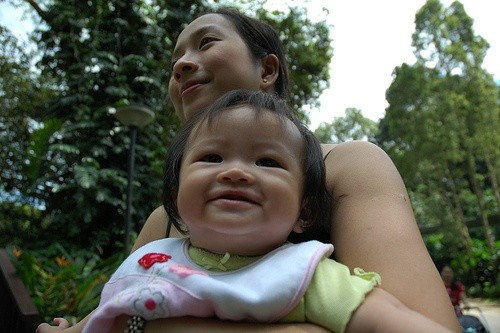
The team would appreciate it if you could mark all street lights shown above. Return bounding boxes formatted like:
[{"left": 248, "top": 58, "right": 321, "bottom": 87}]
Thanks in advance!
[{"left": 115, "top": 102, "right": 156, "bottom": 259}]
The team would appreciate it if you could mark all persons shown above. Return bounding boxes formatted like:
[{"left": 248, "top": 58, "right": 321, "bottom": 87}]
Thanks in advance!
[
  {"left": 36, "top": 90, "right": 464, "bottom": 333},
  {"left": 439, "top": 266, "right": 471, "bottom": 319},
  {"left": 129, "top": 7, "right": 464, "bottom": 333}
]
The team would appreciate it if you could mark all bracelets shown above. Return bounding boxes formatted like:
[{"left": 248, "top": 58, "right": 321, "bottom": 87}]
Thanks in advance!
[{"left": 125, "top": 317, "right": 143, "bottom": 333}]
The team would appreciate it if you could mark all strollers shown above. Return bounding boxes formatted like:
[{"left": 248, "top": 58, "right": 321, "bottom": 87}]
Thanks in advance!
[{"left": 451, "top": 305, "right": 491, "bottom": 333}]
